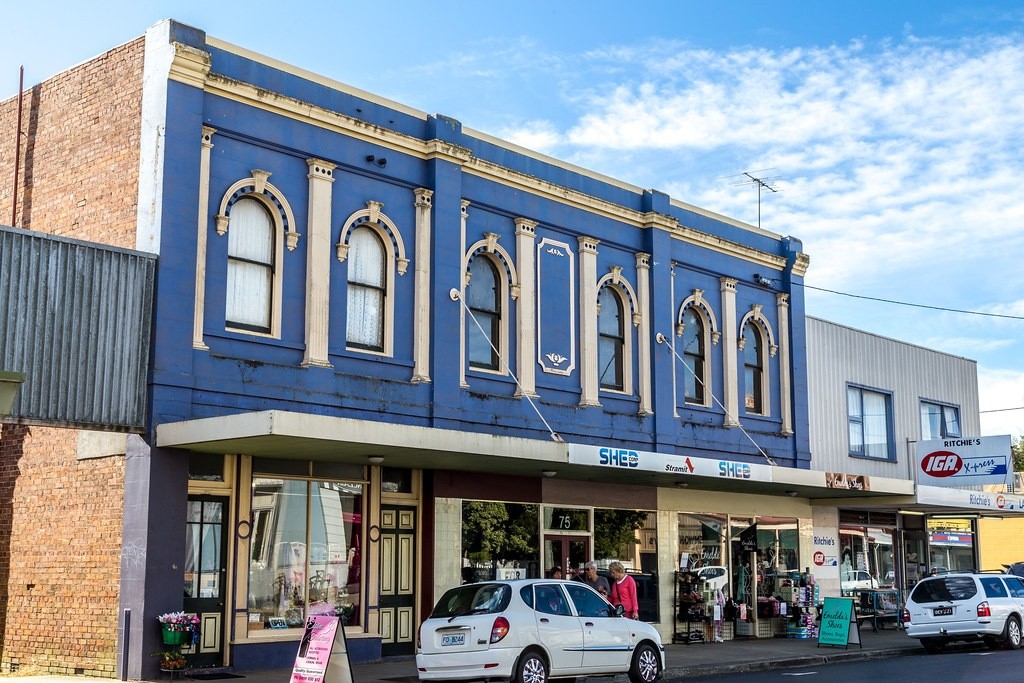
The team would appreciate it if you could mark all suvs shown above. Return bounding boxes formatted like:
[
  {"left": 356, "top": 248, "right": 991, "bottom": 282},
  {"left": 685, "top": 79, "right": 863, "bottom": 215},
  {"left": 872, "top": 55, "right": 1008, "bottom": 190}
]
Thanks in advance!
[{"left": 904, "top": 568, "right": 1024, "bottom": 653}]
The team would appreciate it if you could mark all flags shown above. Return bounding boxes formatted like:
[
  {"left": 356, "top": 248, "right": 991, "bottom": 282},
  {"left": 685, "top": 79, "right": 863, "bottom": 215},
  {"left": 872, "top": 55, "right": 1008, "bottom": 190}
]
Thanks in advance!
[
  {"left": 701, "top": 524, "right": 721, "bottom": 553},
  {"left": 739, "top": 523, "right": 758, "bottom": 553}
]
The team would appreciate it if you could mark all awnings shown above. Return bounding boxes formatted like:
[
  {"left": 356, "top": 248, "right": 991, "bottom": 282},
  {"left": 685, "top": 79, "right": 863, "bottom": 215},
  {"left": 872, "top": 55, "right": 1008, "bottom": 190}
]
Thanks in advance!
[
  {"left": 684, "top": 512, "right": 797, "bottom": 543},
  {"left": 866, "top": 528, "right": 893, "bottom": 546}
]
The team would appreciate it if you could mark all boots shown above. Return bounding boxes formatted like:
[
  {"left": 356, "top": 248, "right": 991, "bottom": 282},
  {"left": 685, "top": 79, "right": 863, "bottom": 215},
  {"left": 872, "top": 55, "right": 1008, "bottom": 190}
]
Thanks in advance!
[
  {"left": 791, "top": 606, "right": 807, "bottom": 627},
  {"left": 745, "top": 606, "right": 756, "bottom": 623}
]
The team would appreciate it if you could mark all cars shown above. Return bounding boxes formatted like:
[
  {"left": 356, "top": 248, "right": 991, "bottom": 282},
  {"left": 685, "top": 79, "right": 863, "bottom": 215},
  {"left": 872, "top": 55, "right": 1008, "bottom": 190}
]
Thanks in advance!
[
  {"left": 415, "top": 579, "right": 666, "bottom": 683},
  {"left": 689, "top": 566, "right": 728, "bottom": 594},
  {"left": 840, "top": 570, "right": 879, "bottom": 597},
  {"left": 568, "top": 568, "right": 659, "bottom": 622},
  {"left": 1000, "top": 562, "right": 1024, "bottom": 591}
]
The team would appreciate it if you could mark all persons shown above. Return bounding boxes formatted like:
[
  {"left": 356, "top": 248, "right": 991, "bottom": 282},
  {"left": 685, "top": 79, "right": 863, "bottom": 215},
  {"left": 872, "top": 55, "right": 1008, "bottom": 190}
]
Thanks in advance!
[
  {"left": 596, "top": 562, "right": 639, "bottom": 619},
  {"left": 550, "top": 566, "right": 562, "bottom": 579},
  {"left": 583, "top": 560, "right": 611, "bottom": 596},
  {"left": 840, "top": 547, "right": 854, "bottom": 582}
]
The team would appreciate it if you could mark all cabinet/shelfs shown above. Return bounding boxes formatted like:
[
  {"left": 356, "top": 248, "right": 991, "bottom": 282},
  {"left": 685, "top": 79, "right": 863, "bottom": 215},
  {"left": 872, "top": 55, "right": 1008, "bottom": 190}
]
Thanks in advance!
[
  {"left": 842, "top": 588, "right": 900, "bottom": 632},
  {"left": 672, "top": 570, "right": 706, "bottom": 645}
]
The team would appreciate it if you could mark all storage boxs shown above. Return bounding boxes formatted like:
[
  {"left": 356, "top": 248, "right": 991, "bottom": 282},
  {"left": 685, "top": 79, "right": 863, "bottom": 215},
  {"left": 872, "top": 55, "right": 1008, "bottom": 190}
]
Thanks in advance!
[
  {"left": 775, "top": 578, "right": 819, "bottom": 639},
  {"left": 248, "top": 608, "right": 265, "bottom": 630}
]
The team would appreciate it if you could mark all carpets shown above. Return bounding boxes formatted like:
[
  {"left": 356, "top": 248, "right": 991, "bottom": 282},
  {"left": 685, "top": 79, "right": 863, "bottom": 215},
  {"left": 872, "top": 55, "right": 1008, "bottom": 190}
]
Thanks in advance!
[{"left": 185, "top": 673, "right": 246, "bottom": 680}]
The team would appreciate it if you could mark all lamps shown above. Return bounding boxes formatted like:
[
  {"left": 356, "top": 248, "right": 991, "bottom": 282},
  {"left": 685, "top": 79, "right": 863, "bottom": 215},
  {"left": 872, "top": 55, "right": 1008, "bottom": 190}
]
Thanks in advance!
[
  {"left": 676, "top": 483, "right": 688, "bottom": 488},
  {"left": 542, "top": 470, "right": 557, "bottom": 477},
  {"left": 787, "top": 492, "right": 798, "bottom": 497},
  {"left": 368, "top": 455, "right": 384, "bottom": 464}
]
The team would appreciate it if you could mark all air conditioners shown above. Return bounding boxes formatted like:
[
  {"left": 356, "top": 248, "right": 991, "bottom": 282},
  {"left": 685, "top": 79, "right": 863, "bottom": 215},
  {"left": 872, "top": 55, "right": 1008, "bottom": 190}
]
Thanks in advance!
[{"left": 983, "top": 474, "right": 1022, "bottom": 493}]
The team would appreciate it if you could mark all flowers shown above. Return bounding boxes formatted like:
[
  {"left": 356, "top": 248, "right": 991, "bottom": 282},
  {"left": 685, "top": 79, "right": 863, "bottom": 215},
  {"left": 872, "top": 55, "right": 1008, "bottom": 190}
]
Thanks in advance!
[{"left": 151, "top": 651, "right": 187, "bottom": 669}]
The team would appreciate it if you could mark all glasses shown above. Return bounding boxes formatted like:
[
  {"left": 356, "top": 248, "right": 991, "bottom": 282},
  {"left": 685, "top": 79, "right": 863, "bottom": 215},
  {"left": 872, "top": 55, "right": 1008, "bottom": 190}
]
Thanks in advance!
[{"left": 584, "top": 567, "right": 595, "bottom": 572}]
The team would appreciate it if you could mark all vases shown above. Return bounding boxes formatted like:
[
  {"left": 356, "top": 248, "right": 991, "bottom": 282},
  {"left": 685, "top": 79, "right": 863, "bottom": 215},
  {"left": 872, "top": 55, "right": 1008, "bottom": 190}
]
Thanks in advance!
[{"left": 170, "top": 672, "right": 179, "bottom": 680}]
